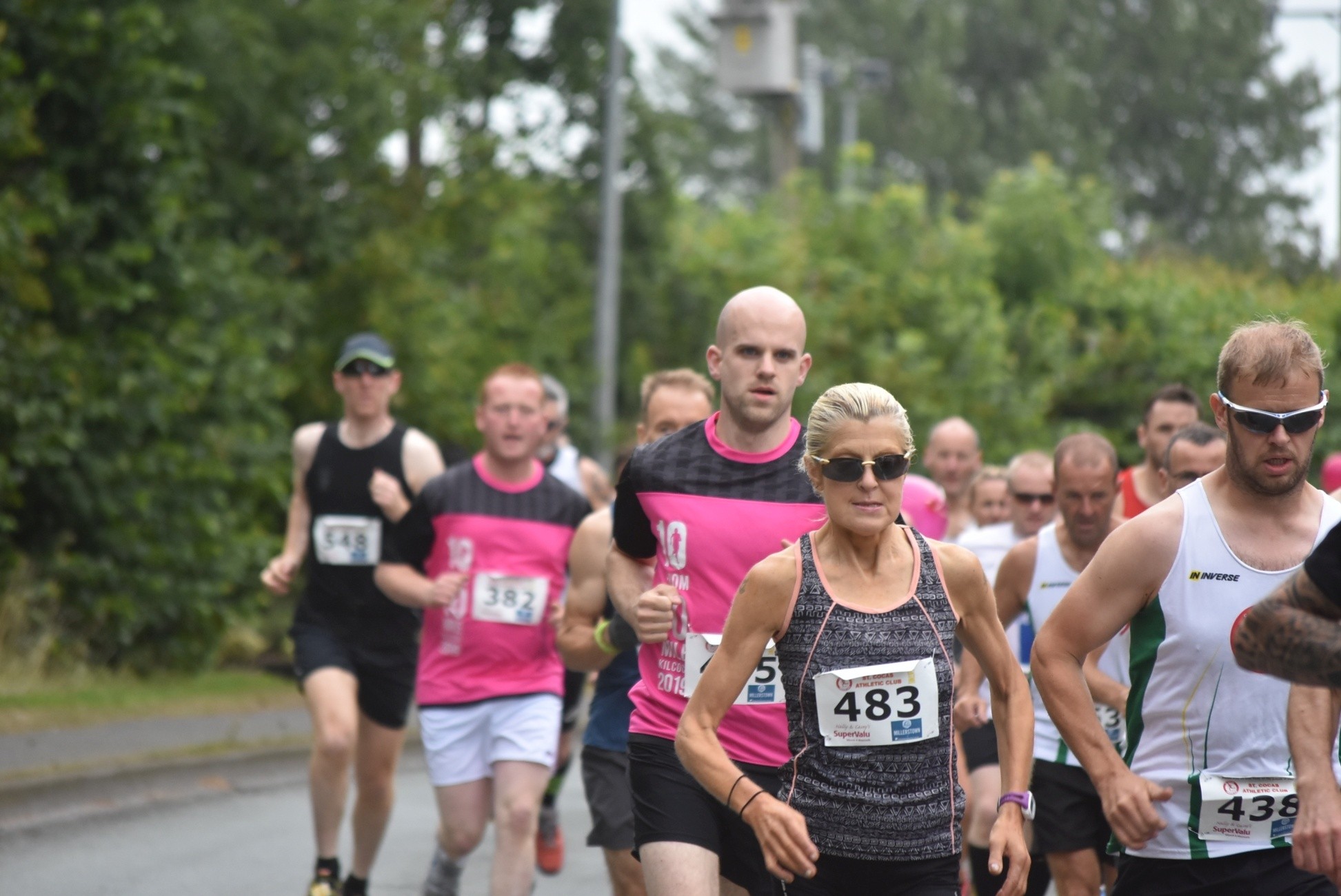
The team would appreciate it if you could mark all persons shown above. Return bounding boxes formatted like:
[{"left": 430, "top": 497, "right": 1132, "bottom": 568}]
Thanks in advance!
[
  {"left": 490, "top": 285, "right": 1341, "bottom": 895},
  {"left": 259, "top": 332, "right": 444, "bottom": 896},
  {"left": 374, "top": 361, "right": 592, "bottom": 895}
]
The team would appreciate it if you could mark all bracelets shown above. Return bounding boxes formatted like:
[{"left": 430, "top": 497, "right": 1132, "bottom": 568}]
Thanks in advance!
[
  {"left": 727, "top": 774, "right": 751, "bottom": 813},
  {"left": 594, "top": 621, "right": 632, "bottom": 661},
  {"left": 737, "top": 789, "right": 771, "bottom": 820}
]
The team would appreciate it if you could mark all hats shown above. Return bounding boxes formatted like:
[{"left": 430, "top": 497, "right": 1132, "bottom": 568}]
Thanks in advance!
[{"left": 334, "top": 332, "right": 398, "bottom": 370}]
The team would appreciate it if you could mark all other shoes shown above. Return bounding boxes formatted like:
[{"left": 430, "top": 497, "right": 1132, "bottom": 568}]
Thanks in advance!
[
  {"left": 421, "top": 853, "right": 462, "bottom": 896},
  {"left": 535, "top": 806, "right": 564, "bottom": 875},
  {"left": 308, "top": 866, "right": 341, "bottom": 896}
]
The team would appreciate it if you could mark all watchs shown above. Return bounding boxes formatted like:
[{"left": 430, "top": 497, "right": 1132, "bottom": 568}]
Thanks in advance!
[{"left": 996, "top": 790, "right": 1038, "bottom": 821}]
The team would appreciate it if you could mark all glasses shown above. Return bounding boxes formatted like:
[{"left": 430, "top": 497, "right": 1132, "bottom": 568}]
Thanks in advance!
[
  {"left": 340, "top": 360, "right": 387, "bottom": 378},
  {"left": 1214, "top": 391, "right": 1328, "bottom": 435},
  {"left": 1015, "top": 491, "right": 1056, "bottom": 505},
  {"left": 811, "top": 449, "right": 914, "bottom": 482}
]
